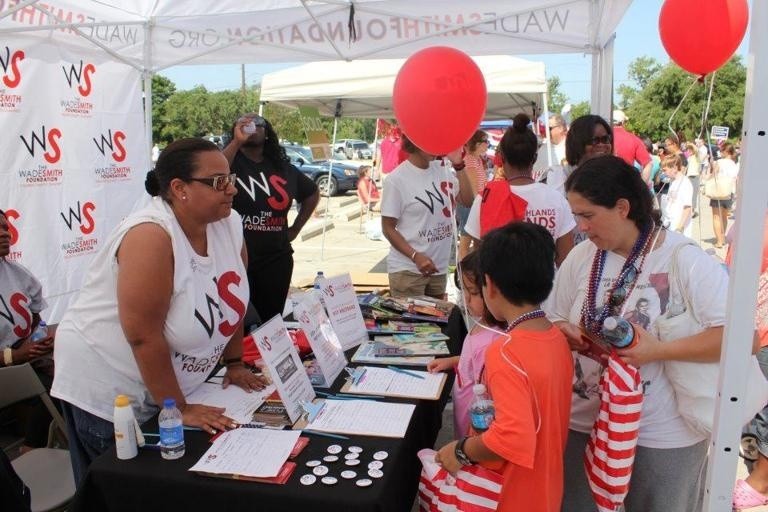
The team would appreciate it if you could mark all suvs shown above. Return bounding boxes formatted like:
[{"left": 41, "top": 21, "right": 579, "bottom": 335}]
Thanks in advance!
[{"left": 343, "top": 138, "right": 373, "bottom": 161}]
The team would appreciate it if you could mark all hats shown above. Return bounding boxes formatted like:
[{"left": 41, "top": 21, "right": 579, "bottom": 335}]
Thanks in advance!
[{"left": 613, "top": 109, "right": 629, "bottom": 124}]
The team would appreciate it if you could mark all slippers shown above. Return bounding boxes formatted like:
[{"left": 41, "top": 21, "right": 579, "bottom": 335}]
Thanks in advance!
[{"left": 732, "top": 479, "right": 768, "bottom": 509}]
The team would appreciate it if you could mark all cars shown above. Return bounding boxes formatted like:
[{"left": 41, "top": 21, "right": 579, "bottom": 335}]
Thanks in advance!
[
  {"left": 200, "top": 133, "right": 223, "bottom": 147},
  {"left": 278, "top": 141, "right": 360, "bottom": 197},
  {"left": 702, "top": 140, "right": 723, "bottom": 163},
  {"left": 326, "top": 138, "right": 352, "bottom": 153},
  {"left": 290, "top": 145, "right": 315, "bottom": 161}
]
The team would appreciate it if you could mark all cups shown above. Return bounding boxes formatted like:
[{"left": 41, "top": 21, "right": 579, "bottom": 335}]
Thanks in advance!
[{"left": 241, "top": 121, "right": 257, "bottom": 135}]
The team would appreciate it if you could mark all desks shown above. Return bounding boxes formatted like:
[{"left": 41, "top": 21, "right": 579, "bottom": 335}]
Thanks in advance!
[{"left": 93, "top": 294, "right": 469, "bottom": 511}]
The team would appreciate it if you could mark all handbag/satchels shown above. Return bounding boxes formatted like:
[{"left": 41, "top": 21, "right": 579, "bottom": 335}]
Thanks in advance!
[
  {"left": 417, "top": 448, "right": 504, "bottom": 512},
  {"left": 657, "top": 242, "right": 768, "bottom": 439},
  {"left": 583, "top": 344, "right": 644, "bottom": 512}
]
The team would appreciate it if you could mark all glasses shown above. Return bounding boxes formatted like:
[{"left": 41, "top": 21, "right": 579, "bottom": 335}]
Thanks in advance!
[
  {"left": 588, "top": 135, "right": 610, "bottom": 144},
  {"left": 238, "top": 116, "right": 267, "bottom": 127},
  {"left": 193, "top": 173, "right": 237, "bottom": 191}
]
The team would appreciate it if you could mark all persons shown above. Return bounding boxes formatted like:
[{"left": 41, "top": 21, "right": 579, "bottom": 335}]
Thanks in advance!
[
  {"left": 221, "top": 112, "right": 319, "bottom": 328},
  {"left": 0, "top": 210, "right": 63, "bottom": 490},
  {"left": 358, "top": 112, "right": 767, "bottom": 511},
  {"left": 49, "top": 137, "right": 272, "bottom": 490}
]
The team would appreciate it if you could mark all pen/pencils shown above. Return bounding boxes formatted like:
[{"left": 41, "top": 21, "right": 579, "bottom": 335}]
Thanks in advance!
[
  {"left": 295, "top": 429, "right": 349, "bottom": 439},
  {"left": 387, "top": 365, "right": 426, "bottom": 379},
  {"left": 315, "top": 391, "right": 385, "bottom": 401}
]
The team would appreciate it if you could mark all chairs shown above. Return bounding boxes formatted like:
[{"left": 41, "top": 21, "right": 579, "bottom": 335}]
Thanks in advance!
[
  {"left": 0, "top": 362, "right": 97, "bottom": 512},
  {"left": 357, "top": 190, "right": 376, "bottom": 238}
]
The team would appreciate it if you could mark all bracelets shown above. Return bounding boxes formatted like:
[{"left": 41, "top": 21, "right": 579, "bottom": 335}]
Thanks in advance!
[
  {"left": 3, "top": 348, "right": 13, "bottom": 366},
  {"left": 222, "top": 353, "right": 244, "bottom": 368}
]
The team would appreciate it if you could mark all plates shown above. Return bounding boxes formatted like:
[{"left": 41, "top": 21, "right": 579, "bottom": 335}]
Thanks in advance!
[{"left": 241, "top": 329, "right": 311, "bottom": 365}]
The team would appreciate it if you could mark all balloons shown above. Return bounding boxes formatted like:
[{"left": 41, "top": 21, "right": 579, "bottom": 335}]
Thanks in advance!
[
  {"left": 659, "top": 0, "right": 749, "bottom": 84},
  {"left": 390, "top": 46, "right": 488, "bottom": 156}
]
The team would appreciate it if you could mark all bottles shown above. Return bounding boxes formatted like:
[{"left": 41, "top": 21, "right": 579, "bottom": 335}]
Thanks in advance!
[
  {"left": 158, "top": 399, "right": 186, "bottom": 461},
  {"left": 468, "top": 384, "right": 496, "bottom": 434},
  {"left": 113, "top": 394, "right": 138, "bottom": 461},
  {"left": 313, "top": 271, "right": 325, "bottom": 289},
  {"left": 31, "top": 321, "right": 48, "bottom": 342},
  {"left": 601, "top": 316, "right": 635, "bottom": 348}
]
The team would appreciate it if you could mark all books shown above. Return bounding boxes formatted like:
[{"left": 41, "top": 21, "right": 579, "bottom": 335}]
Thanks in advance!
[{"left": 345, "top": 281, "right": 463, "bottom": 356}]
